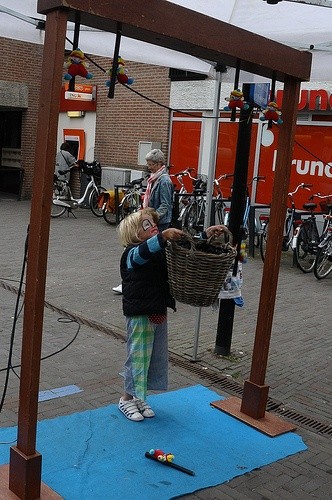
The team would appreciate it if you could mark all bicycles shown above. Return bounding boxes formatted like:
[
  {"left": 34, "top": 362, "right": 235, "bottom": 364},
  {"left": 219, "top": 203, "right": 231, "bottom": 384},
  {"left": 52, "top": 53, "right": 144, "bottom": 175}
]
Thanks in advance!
[
  {"left": 223, "top": 175, "right": 266, "bottom": 250},
  {"left": 102, "top": 164, "right": 235, "bottom": 237},
  {"left": 292, "top": 192, "right": 332, "bottom": 273},
  {"left": 258, "top": 183, "right": 313, "bottom": 264},
  {"left": 313, "top": 204, "right": 332, "bottom": 280},
  {"left": 51, "top": 160, "right": 110, "bottom": 218}
]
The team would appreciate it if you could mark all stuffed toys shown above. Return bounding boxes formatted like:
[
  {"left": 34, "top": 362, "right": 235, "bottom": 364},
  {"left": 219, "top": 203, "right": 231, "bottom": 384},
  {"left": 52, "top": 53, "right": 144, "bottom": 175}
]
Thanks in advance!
[
  {"left": 259, "top": 101, "right": 284, "bottom": 125},
  {"left": 224, "top": 89, "right": 249, "bottom": 111},
  {"left": 62, "top": 48, "right": 94, "bottom": 81},
  {"left": 149, "top": 448, "right": 175, "bottom": 463},
  {"left": 105, "top": 56, "right": 135, "bottom": 87}
]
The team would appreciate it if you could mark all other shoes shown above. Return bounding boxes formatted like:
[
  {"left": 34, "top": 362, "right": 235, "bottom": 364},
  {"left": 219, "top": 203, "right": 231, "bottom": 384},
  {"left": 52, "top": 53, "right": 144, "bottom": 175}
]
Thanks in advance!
[
  {"left": 119, "top": 397, "right": 143, "bottom": 421},
  {"left": 112, "top": 284, "right": 122, "bottom": 293},
  {"left": 134, "top": 396, "right": 155, "bottom": 418}
]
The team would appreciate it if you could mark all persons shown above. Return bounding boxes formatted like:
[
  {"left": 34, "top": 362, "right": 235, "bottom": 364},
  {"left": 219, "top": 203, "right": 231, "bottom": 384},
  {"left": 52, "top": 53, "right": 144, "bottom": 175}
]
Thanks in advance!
[
  {"left": 55, "top": 142, "right": 78, "bottom": 194},
  {"left": 111, "top": 148, "right": 175, "bottom": 295},
  {"left": 114, "top": 210, "right": 229, "bottom": 421}
]
